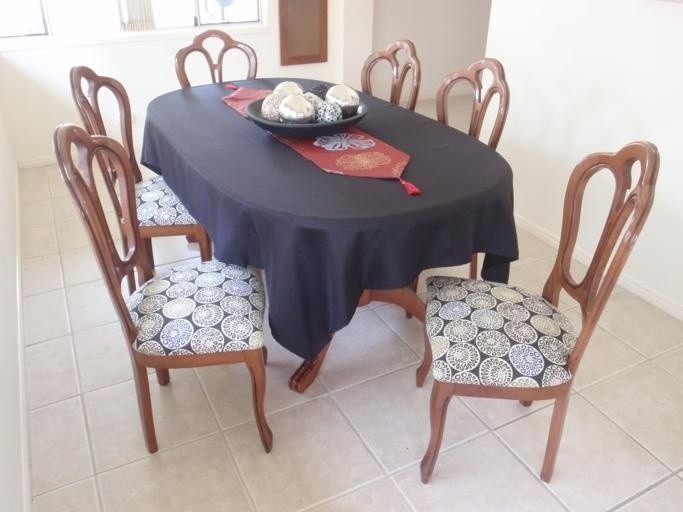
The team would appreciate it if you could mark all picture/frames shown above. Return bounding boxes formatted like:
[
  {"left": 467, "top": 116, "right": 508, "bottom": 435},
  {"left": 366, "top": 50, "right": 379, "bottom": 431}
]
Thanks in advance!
[{"left": 277, "top": 0, "right": 327, "bottom": 67}]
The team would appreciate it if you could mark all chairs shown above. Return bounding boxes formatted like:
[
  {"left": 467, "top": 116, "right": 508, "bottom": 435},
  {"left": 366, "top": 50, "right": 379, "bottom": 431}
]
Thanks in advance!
[
  {"left": 52, "top": 122, "right": 276, "bottom": 457},
  {"left": 175, "top": 30, "right": 256, "bottom": 88},
  {"left": 405, "top": 56, "right": 510, "bottom": 323},
  {"left": 411, "top": 140, "right": 661, "bottom": 486},
  {"left": 361, "top": 39, "right": 421, "bottom": 110},
  {"left": 68, "top": 64, "right": 210, "bottom": 291}
]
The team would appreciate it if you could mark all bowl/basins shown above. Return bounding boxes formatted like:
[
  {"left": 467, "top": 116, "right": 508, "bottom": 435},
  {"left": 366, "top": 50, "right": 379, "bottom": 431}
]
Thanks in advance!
[{"left": 246, "top": 98, "right": 368, "bottom": 138}]
